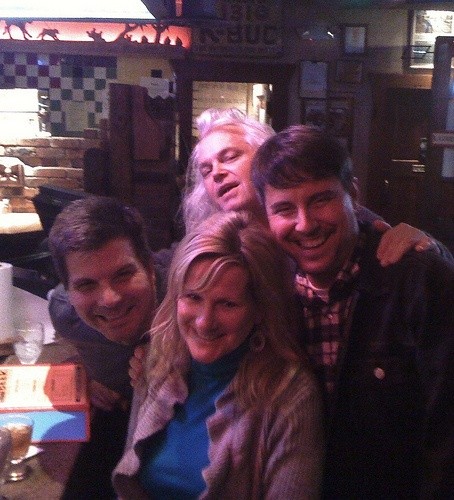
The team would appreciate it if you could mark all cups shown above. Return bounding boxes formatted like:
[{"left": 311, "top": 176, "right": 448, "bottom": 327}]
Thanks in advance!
[
  {"left": 12, "top": 319, "right": 44, "bottom": 365},
  {"left": 0, "top": 414, "right": 34, "bottom": 482},
  {"left": 0, "top": 427, "right": 11, "bottom": 485}
]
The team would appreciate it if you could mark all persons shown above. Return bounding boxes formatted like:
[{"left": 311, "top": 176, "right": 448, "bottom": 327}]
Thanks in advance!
[
  {"left": 181, "top": 105, "right": 454, "bottom": 267},
  {"left": 110, "top": 215, "right": 327, "bottom": 500},
  {"left": 130, "top": 126, "right": 454, "bottom": 500},
  {"left": 44, "top": 197, "right": 182, "bottom": 466}
]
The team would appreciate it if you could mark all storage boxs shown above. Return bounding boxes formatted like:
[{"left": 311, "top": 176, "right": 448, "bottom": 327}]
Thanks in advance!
[{"left": 1, "top": 362, "right": 92, "bottom": 443}]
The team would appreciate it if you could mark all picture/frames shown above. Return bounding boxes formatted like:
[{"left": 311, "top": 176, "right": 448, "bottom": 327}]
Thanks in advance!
[
  {"left": 342, "top": 23, "right": 367, "bottom": 56},
  {"left": 334, "top": 58, "right": 362, "bottom": 83}
]
